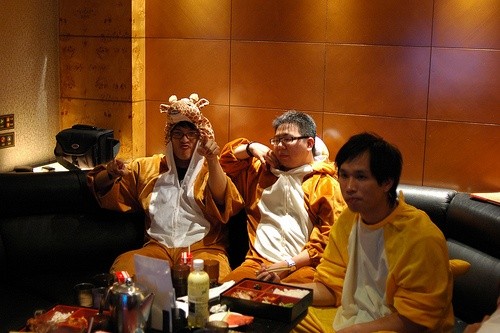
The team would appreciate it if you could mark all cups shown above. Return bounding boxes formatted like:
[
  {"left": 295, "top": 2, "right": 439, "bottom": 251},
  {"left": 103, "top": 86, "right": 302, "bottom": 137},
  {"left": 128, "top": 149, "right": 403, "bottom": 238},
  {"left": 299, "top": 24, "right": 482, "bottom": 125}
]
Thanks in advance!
[
  {"left": 204, "top": 259, "right": 220, "bottom": 306},
  {"left": 75, "top": 282, "right": 95, "bottom": 309},
  {"left": 170, "top": 264, "right": 190, "bottom": 302}
]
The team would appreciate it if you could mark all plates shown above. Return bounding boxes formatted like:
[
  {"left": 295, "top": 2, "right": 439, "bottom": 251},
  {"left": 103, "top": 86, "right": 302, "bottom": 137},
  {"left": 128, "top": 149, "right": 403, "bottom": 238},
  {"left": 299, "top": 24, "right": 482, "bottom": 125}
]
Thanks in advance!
[{"left": 208, "top": 311, "right": 242, "bottom": 328}]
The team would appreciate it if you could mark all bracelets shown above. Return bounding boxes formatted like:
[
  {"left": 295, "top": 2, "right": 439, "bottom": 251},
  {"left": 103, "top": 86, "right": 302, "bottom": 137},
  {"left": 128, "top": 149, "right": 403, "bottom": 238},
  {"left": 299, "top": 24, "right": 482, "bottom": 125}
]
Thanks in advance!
[{"left": 246, "top": 142, "right": 254, "bottom": 158}]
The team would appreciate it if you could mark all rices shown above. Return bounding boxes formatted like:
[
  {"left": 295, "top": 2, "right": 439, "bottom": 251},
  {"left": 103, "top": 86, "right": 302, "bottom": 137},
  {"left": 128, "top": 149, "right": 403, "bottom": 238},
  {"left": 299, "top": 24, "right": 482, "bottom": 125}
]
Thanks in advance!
[
  {"left": 272, "top": 288, "right": 309, "bottom": 298},
  {"left": 51, "top": 311, "right": 74, "bottom": 322}
]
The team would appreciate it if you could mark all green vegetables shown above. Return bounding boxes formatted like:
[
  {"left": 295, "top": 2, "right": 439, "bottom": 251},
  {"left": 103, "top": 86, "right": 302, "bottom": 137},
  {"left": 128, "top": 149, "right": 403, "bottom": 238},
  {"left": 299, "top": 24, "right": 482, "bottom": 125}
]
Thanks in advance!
[{"left": 282, "top": 303, "right": 294, "bottom": 307}]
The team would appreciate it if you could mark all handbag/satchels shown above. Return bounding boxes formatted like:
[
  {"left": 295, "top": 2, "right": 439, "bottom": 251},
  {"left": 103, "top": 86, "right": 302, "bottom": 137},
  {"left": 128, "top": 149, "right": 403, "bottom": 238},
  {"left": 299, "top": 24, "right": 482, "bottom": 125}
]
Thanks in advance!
[{"left": 53, "top": 124, "right": 119, "bottom": 166}]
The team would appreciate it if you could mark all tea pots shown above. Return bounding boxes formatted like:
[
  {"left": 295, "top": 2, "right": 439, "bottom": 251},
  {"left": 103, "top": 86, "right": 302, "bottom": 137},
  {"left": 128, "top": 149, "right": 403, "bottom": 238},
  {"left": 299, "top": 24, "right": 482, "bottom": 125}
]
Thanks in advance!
[{"left": 103, "top": 277, "right": 155, "bottom": 332}]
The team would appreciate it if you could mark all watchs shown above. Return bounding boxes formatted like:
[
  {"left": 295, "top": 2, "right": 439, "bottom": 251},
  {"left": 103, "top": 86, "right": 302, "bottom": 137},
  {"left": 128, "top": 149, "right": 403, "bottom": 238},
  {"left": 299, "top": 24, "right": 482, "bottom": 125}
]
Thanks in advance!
[{"left": 285, "top": 259, "right": 297, "bottom": 274}]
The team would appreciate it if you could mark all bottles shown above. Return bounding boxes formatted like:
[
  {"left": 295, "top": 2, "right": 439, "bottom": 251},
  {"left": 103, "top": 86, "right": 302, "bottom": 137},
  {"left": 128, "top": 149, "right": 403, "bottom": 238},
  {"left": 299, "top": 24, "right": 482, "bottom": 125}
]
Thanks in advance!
[{"left": 186, "top": 259, "right": 210, "bottom": 330}]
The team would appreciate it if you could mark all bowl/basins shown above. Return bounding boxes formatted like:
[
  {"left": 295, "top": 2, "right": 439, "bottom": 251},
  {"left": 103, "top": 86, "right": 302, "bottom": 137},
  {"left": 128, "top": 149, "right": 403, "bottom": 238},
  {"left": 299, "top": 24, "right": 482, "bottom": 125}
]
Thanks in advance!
[
  {"left": 219, "top": 277, "right": 314, "bottom": 321},
  {"left": 27, "top": 305, "right": 99, "bottom": 333}
]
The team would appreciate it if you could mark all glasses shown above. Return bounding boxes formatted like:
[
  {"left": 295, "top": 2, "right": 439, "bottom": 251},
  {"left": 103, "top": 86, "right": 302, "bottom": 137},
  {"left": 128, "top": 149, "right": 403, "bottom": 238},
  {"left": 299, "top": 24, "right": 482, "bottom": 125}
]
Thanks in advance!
[
  {"left": 171, "top": 132, "right": 198, "bottom": 139},
  {"left": 270, "top": 136, "right": 310, "bottom": 145}
]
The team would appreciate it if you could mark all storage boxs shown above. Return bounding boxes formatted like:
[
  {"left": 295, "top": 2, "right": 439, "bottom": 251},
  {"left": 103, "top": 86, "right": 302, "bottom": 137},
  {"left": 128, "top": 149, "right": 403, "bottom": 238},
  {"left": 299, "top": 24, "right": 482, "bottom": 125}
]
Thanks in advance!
[
  {"left": 28, "top": 304, "right": 110, "bottom": 333},
  {"left": 220, "top": 278, "right": 313, "bottom": 324}
]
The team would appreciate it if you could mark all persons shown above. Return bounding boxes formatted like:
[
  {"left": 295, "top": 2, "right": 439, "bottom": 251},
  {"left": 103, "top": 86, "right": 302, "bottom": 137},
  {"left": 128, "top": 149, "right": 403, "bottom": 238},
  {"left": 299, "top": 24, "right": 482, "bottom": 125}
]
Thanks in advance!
[
  {"left": 92, "top": 93, "right": 244, "bottom": 288},
  {"left": 220, "top": 110, "right": 349, "bottom": 285},
  {"left": 256, "top": 133, "right": 455, "bottom": 333}
]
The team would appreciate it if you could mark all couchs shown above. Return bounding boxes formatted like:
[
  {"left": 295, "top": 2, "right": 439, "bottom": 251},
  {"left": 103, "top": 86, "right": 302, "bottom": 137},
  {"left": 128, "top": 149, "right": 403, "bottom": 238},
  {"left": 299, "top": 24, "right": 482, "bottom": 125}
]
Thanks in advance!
[{"left": 0, "top": 169, "right": 500, "bottom": 333}]
